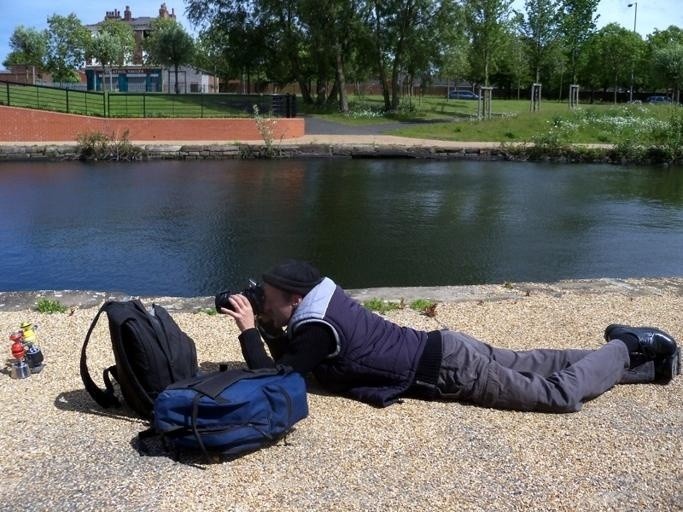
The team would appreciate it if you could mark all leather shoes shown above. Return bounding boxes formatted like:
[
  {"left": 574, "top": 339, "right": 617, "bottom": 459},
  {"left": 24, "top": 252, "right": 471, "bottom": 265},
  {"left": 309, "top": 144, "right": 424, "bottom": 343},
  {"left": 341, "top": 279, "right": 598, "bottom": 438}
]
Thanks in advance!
[
  {"left": 605, "top": 324, "right": 676, "bottom": 356},
  {"left": 655, "top": 346, "right": 681, "bottom": 385}
]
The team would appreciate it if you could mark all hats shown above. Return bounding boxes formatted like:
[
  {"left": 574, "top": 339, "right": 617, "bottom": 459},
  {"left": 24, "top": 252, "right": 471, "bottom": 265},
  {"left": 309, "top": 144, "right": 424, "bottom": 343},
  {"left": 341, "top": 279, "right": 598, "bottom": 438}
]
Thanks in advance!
[{"left": 261, "top": 259, "right": 322, "bottom": 295}]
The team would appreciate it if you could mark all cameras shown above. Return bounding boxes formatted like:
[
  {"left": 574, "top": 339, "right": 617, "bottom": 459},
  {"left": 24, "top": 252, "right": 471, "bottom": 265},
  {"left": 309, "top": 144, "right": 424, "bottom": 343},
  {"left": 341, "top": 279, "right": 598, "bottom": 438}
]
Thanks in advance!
[{"left": 214, "top": 283, "right": 263, "bottom": 316}]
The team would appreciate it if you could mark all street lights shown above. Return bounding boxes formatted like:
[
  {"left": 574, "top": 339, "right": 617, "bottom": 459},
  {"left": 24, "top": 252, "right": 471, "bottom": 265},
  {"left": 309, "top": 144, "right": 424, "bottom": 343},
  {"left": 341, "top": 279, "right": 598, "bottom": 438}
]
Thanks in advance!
[{"left": 627, "top": 1, "right": 637, "bottom": 32}]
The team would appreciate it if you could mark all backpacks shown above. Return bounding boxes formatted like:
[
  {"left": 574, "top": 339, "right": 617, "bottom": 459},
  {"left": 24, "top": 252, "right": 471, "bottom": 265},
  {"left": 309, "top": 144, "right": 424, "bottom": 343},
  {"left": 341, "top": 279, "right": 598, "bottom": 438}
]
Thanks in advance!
[
  {"left": 105, "top": 300, "right": 198, "bottom": 419},
  {"left": 154, "top": 366, "right": 309, "bottom": 457}
]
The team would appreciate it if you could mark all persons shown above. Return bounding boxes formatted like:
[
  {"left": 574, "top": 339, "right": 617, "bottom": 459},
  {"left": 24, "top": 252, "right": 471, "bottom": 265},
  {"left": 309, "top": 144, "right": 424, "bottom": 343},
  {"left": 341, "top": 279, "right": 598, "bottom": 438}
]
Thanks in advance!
[{"left": 221, "top": 259, "right": 682, "bottom": 414}]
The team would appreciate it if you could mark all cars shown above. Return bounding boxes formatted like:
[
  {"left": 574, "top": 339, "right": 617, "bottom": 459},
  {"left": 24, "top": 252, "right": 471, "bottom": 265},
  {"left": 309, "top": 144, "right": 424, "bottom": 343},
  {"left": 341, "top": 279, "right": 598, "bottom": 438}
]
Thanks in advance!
[{"left": 450, "top": 90, "right": 484, "bottom": 100}]
